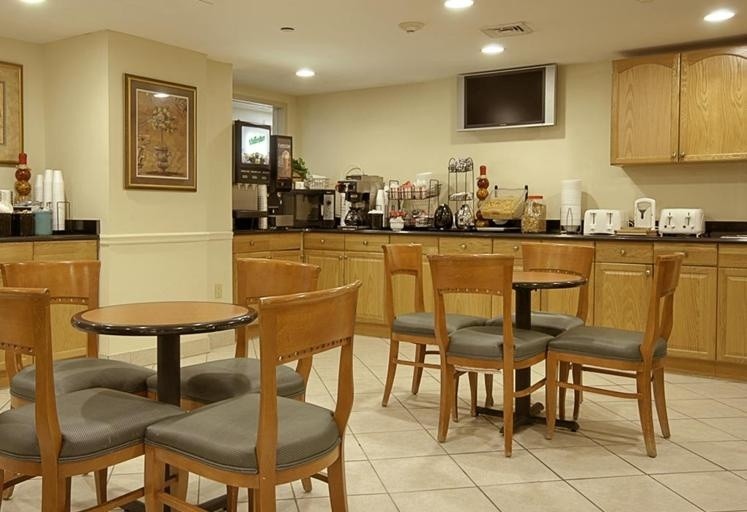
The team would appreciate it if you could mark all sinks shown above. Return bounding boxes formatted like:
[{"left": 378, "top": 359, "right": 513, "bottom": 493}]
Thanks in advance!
[{"left": 719, "top": 233, "right": 747, "bottom": 240}]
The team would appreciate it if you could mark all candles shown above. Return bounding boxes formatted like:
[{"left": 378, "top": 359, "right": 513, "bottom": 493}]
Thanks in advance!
[{"left": 19, "top": 153, "right": 27, "bottom": 164}]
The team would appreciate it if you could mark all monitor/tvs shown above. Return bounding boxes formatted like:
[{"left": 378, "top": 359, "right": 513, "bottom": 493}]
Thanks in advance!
[{"left": 457, "top": 63, "right": 557, "bottom": 132}]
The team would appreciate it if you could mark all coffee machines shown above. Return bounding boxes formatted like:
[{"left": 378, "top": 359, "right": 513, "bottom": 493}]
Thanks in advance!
[
  {"left": 232, "top": 120, "right": 271, "bottom": 231},
  {"left": 335, "top": 175, "right": 383, "bottom": 231},
  {"left": 269, "top": 135, "right": 293, "bottom": 229}
]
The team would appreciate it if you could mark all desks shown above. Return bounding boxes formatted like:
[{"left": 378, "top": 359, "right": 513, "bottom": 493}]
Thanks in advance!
[
  {"left": 475, "top": 270, "right": 589, "bottom": 433},
  {"left": 71, "top": 301, "right": 258, "bottom": 512}
]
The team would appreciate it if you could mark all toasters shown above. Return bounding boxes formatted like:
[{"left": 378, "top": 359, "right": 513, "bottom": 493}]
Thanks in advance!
[
  {"left": 581, "top": 209, "right": 621, "bottom": 237},
  {"left": 658, "top": 208, "right": 705, "bottom": 237}
]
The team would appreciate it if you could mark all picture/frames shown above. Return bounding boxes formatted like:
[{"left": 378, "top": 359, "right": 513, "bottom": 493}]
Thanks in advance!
[
  {"left": 0, "top": 60, "right": 24, "bottom": 164},
  {"left": 124, "top": 73, "right": 198, "bottom": 191}
]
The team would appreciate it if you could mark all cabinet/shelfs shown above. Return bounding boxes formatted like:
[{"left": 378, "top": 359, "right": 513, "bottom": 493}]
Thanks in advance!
[
  {"left": 232, "top": 227, "right": 747, "bottom": 382},
  {"left": 0, "top": 240, "right": 98, "bottom": 377},
  {"left": 610, "top": 42, "right": 746, "bottom": 165}
]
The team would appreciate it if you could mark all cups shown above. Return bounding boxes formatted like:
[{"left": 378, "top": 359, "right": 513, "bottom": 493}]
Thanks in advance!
[
  {"left": 33, "top": 168, "right": 65, "bottom": 232},
  {"left": 310, "top": 175, "right": 331, "bottom": 190},
  {"left": 367, "top": 212, "right": 383, "bottom": 231},
  {"left": 295, "top": 181, "right": 303, "bottom": 190},
  {"left": 375, "top": 189, "right": 388, "bottom": 229},
  {"left": 255, "top": 184, "right": 267, "bottom": 229}
]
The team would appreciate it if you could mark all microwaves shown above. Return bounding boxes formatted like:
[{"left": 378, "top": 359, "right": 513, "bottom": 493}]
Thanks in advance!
[{"left": 282, "top": 189, "right": 334, "bottom": 228}]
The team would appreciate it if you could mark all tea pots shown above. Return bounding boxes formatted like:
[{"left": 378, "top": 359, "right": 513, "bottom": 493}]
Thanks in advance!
[
  {"left": 432, "top": 204, "right": 452, "bottom": 230},
  {"left": 410, "top": 210, "right": 430, "bottom": 228},
  {"left": 455, "top": 204, "right": 471, "bottom": 229}
]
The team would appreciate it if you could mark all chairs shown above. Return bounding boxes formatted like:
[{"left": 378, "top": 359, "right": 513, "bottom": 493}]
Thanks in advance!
[
  {"left": 485, "top": 243, "right": 595, "bottom": 408},
  {"left": 145, "top": 281, "right": 364, "bottom": 512},
  {"left": 0, "top": 287, "right": 190, "bottom": 512},
  {"left": 546, "top": 251, "right": 684, "bottom": 458},
  {"left": 426, "top": 253, "right": 555, "bottom": 458},
  {"left": 377, "top": 244, "right": 489, "bottom": 416},
  {"left": 0, "top": 260, "right": 155, "bottom": 409},
  {"left": 146, "top": 258, "right": 322, "bottom": 492}
]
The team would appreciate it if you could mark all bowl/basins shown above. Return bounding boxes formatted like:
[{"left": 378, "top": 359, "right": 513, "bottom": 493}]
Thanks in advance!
[{"left": 559, "top": 179, "right": 581, "bottom": 233}]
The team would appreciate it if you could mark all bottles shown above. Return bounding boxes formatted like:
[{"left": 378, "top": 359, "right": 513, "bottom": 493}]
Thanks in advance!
[
  {"left": 520, "top": 196, "right": 545, "bottom": 233},
  {"left": 34, "top": 207, "right": 52, "bottom": 237}
]
